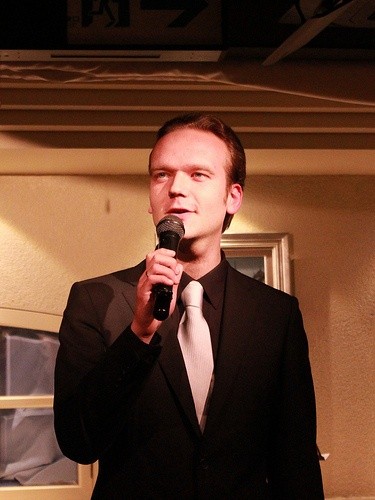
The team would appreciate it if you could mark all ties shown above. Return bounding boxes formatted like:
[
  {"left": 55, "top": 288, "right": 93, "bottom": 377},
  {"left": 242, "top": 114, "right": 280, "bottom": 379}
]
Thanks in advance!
[{"left": 176, "top": 281, "right": 214, "bottom": 425}]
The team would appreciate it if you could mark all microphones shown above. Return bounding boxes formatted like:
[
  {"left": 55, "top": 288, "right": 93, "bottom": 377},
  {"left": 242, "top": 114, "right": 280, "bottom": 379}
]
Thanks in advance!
[{"left": 150, "top": 214, "right": 185, "bottom": 320}]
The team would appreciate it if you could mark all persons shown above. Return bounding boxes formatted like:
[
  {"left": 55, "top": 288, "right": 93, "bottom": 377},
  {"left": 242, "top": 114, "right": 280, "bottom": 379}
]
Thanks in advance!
[{"left": 54, "top": 113, "right": 325, "bottom": 500}]
